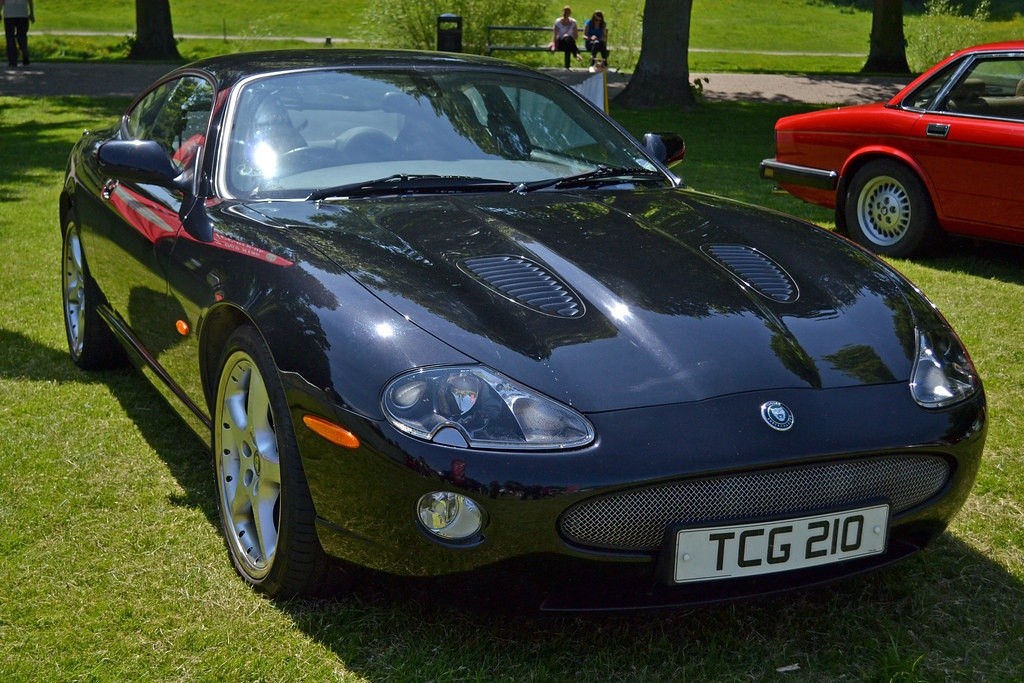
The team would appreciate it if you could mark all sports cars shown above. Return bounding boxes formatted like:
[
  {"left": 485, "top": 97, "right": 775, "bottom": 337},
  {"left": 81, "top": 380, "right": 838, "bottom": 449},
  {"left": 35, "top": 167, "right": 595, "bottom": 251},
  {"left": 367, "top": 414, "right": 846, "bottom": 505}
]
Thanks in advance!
[{"left": 57, "top": 47, "right": 989, "bottom": 616}]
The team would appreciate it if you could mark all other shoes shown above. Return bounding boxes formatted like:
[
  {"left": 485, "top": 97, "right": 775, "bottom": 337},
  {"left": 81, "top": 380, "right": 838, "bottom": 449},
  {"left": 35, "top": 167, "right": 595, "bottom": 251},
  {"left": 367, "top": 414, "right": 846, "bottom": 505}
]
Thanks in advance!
[
  {"left": 10, "top": 62, "right": 16, "bottom": 66},
  {"left": 591, "top": 61, "right": 594, "bottom": 65},
  {"left": 23, "top": 60, "right": 30, "bottom": 65},
  {"left": 603, "top": 60, "right": 607, "bottom": 66},
  {"left": 576, "top": 54, "right": 582, "bottom": 62}
]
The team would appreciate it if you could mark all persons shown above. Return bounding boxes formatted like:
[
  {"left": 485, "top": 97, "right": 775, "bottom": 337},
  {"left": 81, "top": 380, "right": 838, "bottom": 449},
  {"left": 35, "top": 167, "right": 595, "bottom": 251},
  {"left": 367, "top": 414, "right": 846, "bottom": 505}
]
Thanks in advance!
[
  {"left": 549, "top": 6, "right": 583, "bottom": 71},
  {"left": 583, "top": 11, "right": 608, "bottom": 72},
  {"left": 0, "top": 0, "right": 35, "bottom": 69}
]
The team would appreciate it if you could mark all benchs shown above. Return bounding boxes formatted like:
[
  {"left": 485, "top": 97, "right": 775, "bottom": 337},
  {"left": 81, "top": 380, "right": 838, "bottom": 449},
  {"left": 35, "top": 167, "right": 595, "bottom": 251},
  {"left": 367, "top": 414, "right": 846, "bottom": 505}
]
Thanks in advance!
[{"left": 486, "top": 26, "right": 611, "bottom": 59}]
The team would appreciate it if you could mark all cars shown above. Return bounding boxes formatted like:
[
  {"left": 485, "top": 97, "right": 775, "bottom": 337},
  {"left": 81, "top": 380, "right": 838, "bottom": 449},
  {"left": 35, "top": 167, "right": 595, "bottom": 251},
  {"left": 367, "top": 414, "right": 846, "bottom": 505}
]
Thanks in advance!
[{"left": 759, "top": 41, "right": 1023, "bottom": 259}]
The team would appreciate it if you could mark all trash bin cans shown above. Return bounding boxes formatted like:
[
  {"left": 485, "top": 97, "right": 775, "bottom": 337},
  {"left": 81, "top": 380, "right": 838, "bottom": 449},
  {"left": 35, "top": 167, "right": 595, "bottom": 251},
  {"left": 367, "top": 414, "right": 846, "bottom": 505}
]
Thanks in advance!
[{"left": 436, "top": 13, "right": 463, "bottom": 52}]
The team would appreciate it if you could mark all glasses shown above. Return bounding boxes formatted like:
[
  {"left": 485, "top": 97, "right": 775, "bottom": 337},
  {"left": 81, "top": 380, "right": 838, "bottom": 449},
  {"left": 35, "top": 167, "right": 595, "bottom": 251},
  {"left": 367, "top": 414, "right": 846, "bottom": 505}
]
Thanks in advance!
[{"left": 594, "top": 19, "right": 602, "bottom": 22}]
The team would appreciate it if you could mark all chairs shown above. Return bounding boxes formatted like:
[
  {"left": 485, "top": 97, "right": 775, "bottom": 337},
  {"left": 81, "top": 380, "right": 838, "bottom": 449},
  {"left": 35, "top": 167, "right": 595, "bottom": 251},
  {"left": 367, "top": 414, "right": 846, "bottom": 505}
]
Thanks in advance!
[
  {"left": 396, "top": 94, "right": 504, "bottom": 159},
  {"left": 256, "top": 96, "right": 310, "bottom": 176}
]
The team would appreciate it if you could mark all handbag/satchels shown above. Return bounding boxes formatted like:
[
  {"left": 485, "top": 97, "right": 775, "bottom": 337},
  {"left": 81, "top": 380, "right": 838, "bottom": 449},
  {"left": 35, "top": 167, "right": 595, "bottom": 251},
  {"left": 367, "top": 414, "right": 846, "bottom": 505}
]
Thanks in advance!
[{"left": 556, "top": 38, "right": 560, "bottom": 48}]
